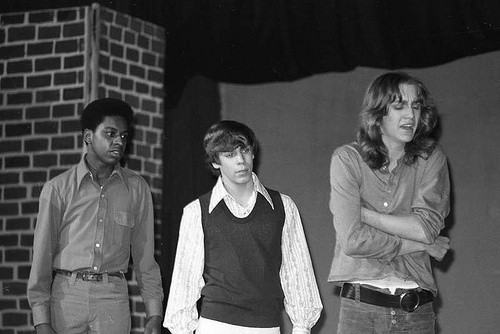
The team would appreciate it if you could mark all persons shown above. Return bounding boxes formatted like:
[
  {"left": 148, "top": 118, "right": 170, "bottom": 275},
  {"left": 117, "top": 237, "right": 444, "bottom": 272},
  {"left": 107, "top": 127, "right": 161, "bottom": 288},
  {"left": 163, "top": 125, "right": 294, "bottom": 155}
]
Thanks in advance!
[
  {"left": 27, "top": 96, "right": 166, "bottom": 334},
  {"left": 161, "top": 119, "right": 324, "bottom": 333},
  {"left": 326, "top": 71, "right": 453, "bottom": 333}
]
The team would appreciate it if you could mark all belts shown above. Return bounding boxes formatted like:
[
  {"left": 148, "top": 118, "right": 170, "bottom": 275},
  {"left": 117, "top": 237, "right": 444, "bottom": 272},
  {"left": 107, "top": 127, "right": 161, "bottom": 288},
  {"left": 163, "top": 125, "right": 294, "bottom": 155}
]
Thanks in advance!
[
  {"left": 341, "top": 282, "right": 434, "bottom": 312},
  {"left": 54, "top": 269, "right": 126, "bottom": 282}
]
